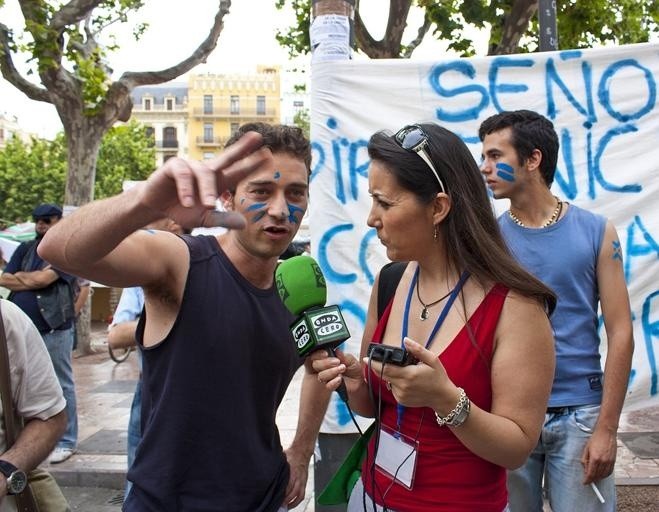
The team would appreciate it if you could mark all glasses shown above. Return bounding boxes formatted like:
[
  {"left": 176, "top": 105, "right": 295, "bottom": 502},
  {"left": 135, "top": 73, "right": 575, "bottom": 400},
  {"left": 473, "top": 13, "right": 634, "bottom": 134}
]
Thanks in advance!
[
  {"left": 394, "top": 124, "right": 447, "bottom": 195},
  {"left": 32, "top": 217, "right": 58, "bottom": 225}
]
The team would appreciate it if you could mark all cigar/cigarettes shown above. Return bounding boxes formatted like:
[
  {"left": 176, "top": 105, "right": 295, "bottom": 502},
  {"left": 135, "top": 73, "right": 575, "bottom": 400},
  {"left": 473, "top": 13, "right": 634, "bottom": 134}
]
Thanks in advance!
[{"left": 590, "top": 481, "right": 605, "bottom": 505}]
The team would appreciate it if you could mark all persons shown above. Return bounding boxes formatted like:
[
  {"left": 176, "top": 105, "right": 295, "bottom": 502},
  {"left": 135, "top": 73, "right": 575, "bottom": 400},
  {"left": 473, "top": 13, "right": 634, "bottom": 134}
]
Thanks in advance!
[
  {"left": 106, "top": 206, "right": 192, "bottom": 505},
  {"left": 35, "top": 122, "right": 334, "bottom": 512},
  {"left": 478, "top": 108, "right": 635, "bottom": 512},
  {"left": 0, "top": 299, "right": 70, "bottom": 512},
  {"left": 304, "top": 122, "right": 556, "bottom": 512},
  {"left": 0, "top": 204, "right": 78, "bottom": 464},
  {"left": 73, "top": 275, "right": 89, "bottom": 351}
]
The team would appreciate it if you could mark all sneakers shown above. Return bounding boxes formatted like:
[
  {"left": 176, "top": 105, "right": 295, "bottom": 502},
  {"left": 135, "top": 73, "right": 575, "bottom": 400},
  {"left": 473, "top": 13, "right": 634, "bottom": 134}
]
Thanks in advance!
[{"left": 47, "top": 446, "right": 78, "bottom": 466}]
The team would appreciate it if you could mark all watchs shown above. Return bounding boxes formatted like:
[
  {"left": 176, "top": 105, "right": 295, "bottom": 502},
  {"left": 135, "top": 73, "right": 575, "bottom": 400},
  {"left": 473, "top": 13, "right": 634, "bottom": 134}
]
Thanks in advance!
[
  {"left": 448, "top": 401, "right": 472, "bottom": 428},
  {"left": 0, "top": 460, "right": 28, "bottom": 497}
]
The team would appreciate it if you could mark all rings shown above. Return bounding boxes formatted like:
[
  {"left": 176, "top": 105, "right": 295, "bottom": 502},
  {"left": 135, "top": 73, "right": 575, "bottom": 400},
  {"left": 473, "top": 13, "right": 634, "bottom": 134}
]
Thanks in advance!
[
  {"left": 385, "top": 381, "right": 392, "bottom": 392},
  {"left": 317, "top": 377, "right": 326, "bottom": 387}
]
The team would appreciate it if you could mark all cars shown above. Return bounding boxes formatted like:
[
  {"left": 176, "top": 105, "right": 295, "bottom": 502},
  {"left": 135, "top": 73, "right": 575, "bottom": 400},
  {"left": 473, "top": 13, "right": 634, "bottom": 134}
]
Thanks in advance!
[{"left": 0, "top": 216, "right": 37, "bottom": 300}]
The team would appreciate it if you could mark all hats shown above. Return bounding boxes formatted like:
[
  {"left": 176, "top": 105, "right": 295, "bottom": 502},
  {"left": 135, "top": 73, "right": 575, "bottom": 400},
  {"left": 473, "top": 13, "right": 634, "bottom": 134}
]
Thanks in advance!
[{"left": 31, "top": 203, "right": 61, "bottom": 217}]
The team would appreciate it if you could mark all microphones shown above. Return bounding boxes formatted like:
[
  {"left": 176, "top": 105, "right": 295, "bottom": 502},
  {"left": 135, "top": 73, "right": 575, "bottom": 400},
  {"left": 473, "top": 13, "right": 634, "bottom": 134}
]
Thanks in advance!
[{"left": 274, "top": 254, "right": 351, "bottom": 404}]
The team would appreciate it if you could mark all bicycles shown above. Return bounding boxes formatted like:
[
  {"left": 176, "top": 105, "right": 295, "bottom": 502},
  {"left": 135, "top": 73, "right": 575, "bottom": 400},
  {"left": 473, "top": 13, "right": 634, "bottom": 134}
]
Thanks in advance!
[{"left": 106, "top": 312, "right": 136, "bottom": 363}]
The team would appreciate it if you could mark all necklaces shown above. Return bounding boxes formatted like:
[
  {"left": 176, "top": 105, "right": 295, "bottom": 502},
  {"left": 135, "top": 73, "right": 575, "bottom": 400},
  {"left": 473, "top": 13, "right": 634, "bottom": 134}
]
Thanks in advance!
[
  {"left": 508, "top": 196, "right": 563, "bottom": 229},
  {"left": 415, "top": 271, "right": 454, "bottom": 321}
]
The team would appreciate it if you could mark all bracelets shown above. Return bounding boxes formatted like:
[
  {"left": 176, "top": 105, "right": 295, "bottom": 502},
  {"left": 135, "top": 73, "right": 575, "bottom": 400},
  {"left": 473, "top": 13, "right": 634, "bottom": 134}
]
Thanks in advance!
[{"left": 433, "top": 384, "right": 471, "bottom": 429}]
